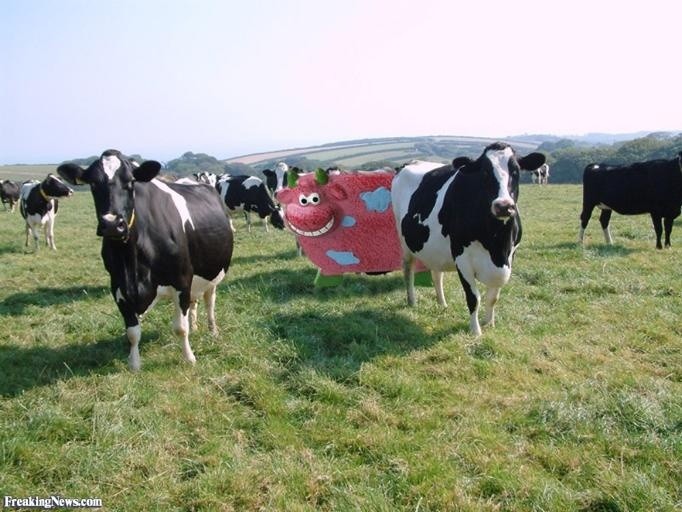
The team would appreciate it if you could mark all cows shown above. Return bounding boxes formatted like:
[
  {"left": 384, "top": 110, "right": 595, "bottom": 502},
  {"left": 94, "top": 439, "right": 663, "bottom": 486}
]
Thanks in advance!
[
  {"left": 391, "top": 142, "right": 546, "bottom": 336},
  {"left": 56, "top": 149, "right": 233, "bottom": 374},
  {"left": 20, "top": 174, "right": 74, "bottom": 251},
  {"left": 193, "top": 172, "right": 218, "bottom": 187},
  {"left": 262, "top": 163, "right": 341, "bottom": 256},
  {"left": 579, "top": 151, "right": 682, "bottom": 250},
  {"left": 531, "top": 163, "right": 549, "bottom": 184},
  {"left": 215, "top": 173, "right": 286, "bottom": 232},
  {"left": 0, "top": 180, "right": 23, "bottom": 213}
]
